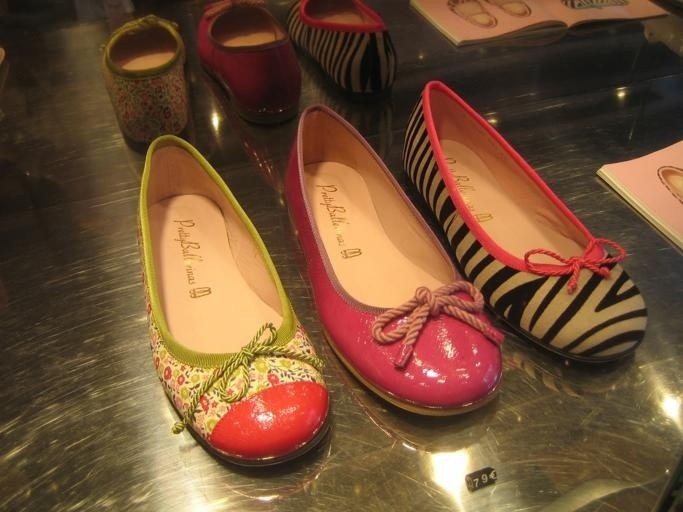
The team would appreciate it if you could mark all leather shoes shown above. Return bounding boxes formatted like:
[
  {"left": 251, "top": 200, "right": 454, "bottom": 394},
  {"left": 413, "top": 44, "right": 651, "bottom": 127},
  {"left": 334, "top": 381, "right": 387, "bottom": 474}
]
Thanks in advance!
[
  {"left": 199, "top": 1, "right": 300, "bottom": 124},
  {"left": 284, "top": 103, "right": 505, "bottom": 417}
]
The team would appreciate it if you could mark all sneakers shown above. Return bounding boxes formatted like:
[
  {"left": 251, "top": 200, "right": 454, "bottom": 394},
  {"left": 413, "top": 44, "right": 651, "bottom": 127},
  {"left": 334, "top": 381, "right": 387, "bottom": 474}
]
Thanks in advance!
[
  {"left": 286, "top": 1, "right": 397, "bottom": 103},
  {"left": 139, "top": 135, "right": 332, "bottom": 467},
  {"left": 402, "top": 81, "right": 647, "bottom": 365},
  {"left": 101, "top": 14, "right": 188, "bottom": 153}
]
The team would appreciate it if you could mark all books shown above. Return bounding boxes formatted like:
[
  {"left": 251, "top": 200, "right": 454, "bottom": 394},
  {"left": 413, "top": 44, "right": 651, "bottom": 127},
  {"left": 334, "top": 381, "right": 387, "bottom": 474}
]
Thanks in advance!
[
  {"left": 408, "top": 0, "right": 669, "bottom": 49},
  {"left": 594, "top": 138, "right": 683, "bottom": 254}
]
